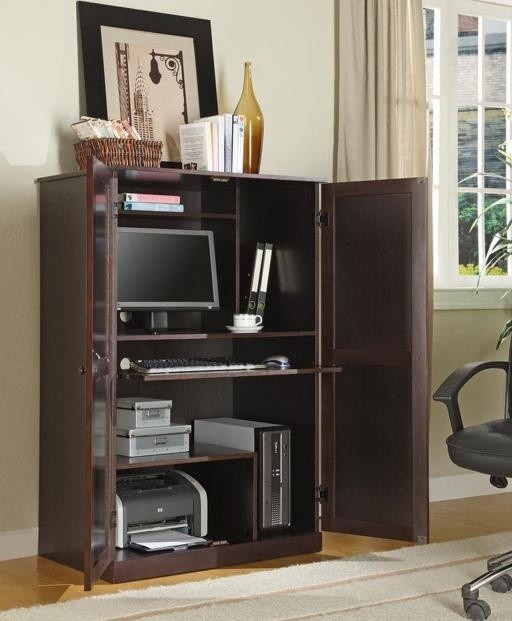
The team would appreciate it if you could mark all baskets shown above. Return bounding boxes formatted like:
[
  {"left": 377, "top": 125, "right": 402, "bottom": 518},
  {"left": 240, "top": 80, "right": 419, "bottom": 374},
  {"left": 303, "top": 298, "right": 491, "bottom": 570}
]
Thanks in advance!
[{"left": 73, "top": 137, "right": 164, "bottom": 172}]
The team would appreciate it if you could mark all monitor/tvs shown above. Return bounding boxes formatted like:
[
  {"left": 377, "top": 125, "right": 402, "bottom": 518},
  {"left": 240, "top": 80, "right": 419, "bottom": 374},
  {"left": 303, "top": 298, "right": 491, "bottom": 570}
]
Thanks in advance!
[{"left": 116, "top": 228, "right": 221, "bottom": 334}]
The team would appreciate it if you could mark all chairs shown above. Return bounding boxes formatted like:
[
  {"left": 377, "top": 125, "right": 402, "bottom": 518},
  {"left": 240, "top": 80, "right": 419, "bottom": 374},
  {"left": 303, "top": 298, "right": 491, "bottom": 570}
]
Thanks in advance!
[{"left": 432, "top": 332, "right": 512, "bottom": 620}]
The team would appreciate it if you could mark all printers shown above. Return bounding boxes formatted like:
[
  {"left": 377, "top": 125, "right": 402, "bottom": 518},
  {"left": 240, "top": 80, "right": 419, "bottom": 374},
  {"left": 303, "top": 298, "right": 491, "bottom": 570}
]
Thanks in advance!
[{"left": 112, "top": 467, "right": 212, "bottom": 561}]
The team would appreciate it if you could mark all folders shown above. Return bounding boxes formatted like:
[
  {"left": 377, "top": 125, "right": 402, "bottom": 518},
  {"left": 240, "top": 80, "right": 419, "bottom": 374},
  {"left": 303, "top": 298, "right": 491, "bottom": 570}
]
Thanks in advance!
[
  {"left": 118, "top": 192, "right": 185, "bottom": 213},
  {"left": 245, "top": 242, "right": 274, "bottom": 326}
]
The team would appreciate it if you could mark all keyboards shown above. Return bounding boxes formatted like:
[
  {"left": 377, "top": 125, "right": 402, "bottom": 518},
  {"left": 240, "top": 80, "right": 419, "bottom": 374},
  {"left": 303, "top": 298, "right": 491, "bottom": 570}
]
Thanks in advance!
[{"left": 130, "top": 358, "right": 263, "bottom": 375}]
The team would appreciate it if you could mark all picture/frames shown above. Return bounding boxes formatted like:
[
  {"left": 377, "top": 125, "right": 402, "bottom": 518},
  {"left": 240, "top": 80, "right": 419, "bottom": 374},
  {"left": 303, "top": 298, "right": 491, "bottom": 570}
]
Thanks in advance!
[{"left": 77, "top": 1, "right": 221, "bottom": 168}]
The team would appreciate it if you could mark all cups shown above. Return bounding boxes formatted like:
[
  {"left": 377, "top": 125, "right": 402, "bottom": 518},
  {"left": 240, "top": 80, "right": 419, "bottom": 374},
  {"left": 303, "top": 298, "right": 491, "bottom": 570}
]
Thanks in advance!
[{"left": 233, "top": 313, "right": 263, "bottom": 327}]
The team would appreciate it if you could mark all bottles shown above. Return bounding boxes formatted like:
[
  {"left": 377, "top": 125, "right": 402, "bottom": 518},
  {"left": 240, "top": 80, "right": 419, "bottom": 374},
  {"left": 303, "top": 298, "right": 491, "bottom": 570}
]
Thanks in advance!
[{"left": 233, "top": 61, "right": 264, "bottom": 174}]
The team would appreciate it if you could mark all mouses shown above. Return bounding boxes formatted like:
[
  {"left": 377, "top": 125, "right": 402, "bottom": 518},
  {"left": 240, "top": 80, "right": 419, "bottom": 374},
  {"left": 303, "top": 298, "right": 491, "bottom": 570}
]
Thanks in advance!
[{"left": 262, "top": 354, "right": 293, "bottom": 367}]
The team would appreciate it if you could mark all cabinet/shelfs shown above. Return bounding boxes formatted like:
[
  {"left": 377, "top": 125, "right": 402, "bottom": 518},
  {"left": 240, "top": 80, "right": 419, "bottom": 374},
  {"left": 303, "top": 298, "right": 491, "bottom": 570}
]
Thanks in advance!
[{"left": 33, "top": 169, "right": 431, "bottom": 593}]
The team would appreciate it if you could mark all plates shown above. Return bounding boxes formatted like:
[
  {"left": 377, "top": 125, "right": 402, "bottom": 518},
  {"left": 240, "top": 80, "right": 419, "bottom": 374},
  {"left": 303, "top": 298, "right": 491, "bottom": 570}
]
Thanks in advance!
[{"left": 226, "top": 326, "right": 264, "bottom": 333}]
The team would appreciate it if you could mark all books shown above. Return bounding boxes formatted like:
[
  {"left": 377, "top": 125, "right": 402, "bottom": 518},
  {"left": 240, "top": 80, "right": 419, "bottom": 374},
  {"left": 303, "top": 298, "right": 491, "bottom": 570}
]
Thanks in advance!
[
  {"left": 94, "top": 194, "right": 107, "bottom": 212},
  {"left": 179, "top": 113, "right": 244, "bottom": 173},
  {"left": 117, "top": 192, "right": 181, "bottom": 204},
  {"left": 71, "top": 115, "right": 143, "bottom": 141},
  {"left": 115, "top": 203, "right": 185, "bottom": 212},
  {"left": 240, "top": 240, "right": 273, "bottom": 327}
]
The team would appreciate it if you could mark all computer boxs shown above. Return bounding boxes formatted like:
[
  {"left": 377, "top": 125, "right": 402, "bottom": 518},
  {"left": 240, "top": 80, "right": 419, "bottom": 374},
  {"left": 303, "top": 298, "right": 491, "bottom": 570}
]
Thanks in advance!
[{"left": 194, "top": 415, "right": 293, "bottom": 532}]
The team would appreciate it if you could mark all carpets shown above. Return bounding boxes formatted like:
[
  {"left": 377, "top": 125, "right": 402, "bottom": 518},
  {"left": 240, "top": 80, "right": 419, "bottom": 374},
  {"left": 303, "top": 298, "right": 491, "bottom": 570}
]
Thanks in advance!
[{"left": 0, "top": 531, "right": 510, "bottom": 621}]
